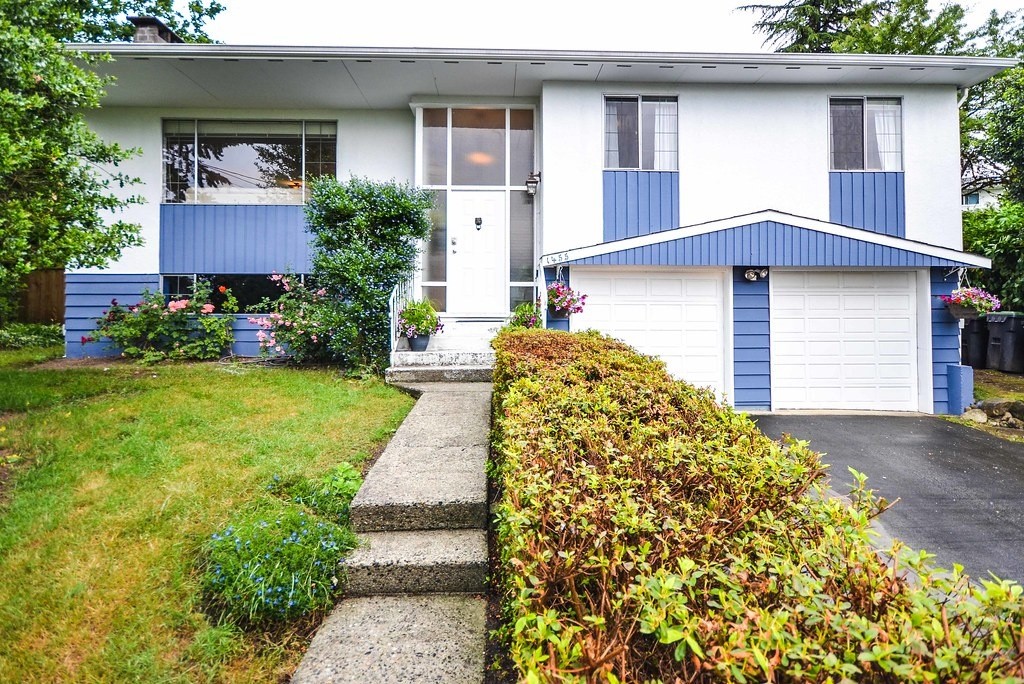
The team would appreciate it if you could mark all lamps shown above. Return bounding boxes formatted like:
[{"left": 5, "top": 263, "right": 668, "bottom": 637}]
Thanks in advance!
[
  {"left": 525, "top": 171, "right": 541, "bottom": 195},
  {"left": 745, "top": 267, "right": 768, "bottom": 280}
]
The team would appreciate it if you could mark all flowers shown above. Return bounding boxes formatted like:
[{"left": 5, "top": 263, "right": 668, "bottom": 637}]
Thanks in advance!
[
  {"left": 546, "top": 281, "right": 587, "bottom": 316},
  {"left": 397, "top": 297, "right": 444, "bottom": 338},
  {"left": 940, "top": 286, "right": 1001, "bottom": 316}
]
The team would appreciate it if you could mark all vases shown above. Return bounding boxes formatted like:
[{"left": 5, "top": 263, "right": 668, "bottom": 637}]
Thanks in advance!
[
  {"left": 946, "top": 301, "right": 980, "bottom": 319},
  {"left": 546, "top": 303, "right": 567, "bottom": 318},
  {"left": 406, "top": 333, "right": 429, "bottom": 351}
]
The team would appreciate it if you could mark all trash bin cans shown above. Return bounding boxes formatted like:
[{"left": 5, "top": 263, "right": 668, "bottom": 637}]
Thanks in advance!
[
  {"left": 986, "top": 311, "right": 1024, "bottom": 373},
  {"left": 961, "top": 310, "right": 990, "bottom": 370}
]
